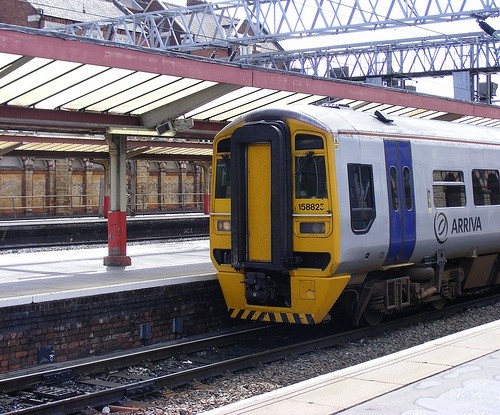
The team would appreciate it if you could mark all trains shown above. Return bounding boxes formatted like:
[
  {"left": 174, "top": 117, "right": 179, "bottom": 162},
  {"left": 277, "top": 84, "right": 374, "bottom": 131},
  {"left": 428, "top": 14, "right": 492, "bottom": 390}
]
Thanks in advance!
[{"left": 206, "top": 102, "right": 500, "bottom": 327}]
